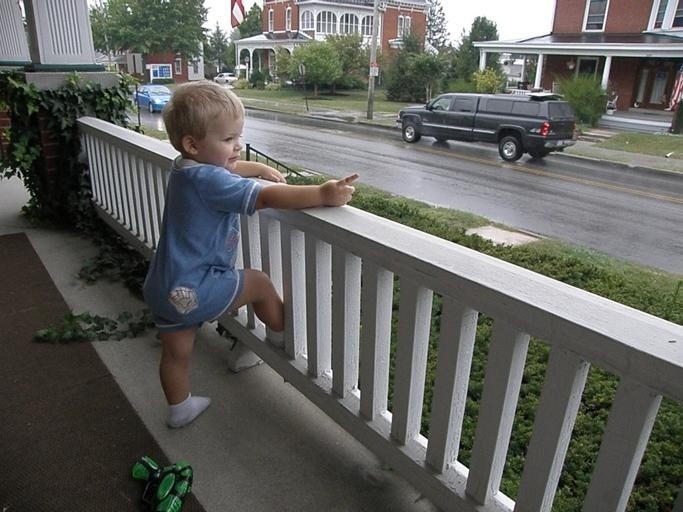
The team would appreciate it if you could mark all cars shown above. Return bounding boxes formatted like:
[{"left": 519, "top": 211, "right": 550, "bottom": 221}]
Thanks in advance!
[
  {"left": 214, "top": 73, "right": 239, "bottom": 84},
  {"left": 133, "top": 85, "right": 173, "bottom": 113}
]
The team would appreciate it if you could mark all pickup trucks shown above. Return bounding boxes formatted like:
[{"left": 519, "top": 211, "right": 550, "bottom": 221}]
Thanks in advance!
[{"left": 394, "top": 93, "right": 576, "bottom": 163}]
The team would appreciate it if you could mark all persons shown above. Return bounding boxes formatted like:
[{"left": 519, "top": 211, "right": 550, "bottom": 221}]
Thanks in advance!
[{"left": 141, "top": 78, "right": 360, "bottom": 429}]
